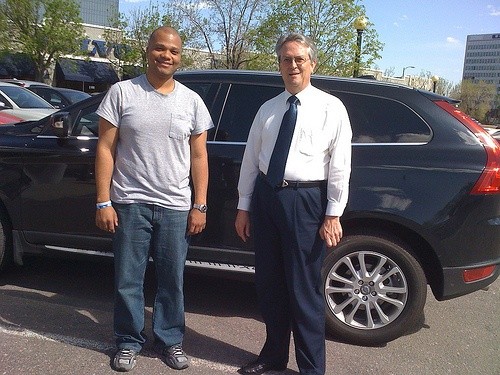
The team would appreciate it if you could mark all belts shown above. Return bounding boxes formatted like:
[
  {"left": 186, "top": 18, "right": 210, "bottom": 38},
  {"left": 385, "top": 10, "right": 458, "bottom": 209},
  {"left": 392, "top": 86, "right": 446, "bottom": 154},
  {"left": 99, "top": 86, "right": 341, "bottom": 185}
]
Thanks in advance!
[{"left": 261, "top": 173, "right": 327, "bottom": 188}]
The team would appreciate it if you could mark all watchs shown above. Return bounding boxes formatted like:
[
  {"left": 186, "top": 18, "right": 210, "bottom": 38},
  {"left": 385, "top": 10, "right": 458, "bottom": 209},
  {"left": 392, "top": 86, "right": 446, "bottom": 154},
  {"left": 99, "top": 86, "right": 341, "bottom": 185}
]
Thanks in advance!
[{"left": 193, "top": 203, "right": 208, "bottom": 213}]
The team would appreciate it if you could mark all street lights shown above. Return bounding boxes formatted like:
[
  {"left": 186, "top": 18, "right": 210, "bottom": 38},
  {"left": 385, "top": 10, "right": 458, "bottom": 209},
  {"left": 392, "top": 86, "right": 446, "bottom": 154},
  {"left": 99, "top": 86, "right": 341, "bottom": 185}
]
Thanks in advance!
[{"left": 354, "top": 16, "right": 367, "bottom": 78}]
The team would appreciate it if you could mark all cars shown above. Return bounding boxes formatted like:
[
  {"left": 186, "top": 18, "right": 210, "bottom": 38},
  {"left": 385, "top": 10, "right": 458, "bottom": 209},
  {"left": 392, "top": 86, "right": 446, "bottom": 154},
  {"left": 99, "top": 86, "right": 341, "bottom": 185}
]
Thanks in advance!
[{"left": 0, "top": 78, "right": 100, "bottom": 138}]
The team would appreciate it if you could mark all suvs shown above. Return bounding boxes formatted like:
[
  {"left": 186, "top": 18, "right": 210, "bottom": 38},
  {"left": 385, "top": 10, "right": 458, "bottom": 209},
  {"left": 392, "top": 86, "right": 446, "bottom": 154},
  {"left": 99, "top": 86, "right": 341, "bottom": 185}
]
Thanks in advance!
[{"left": 0, "top": 68, "right": 500, "bottom": 347}]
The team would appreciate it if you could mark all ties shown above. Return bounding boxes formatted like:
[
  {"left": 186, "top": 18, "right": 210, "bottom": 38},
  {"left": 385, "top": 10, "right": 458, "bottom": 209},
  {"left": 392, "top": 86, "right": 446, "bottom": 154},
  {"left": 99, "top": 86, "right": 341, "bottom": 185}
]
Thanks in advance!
[{"left": 268, "top": 96, "right": 298, "bottom": 186}]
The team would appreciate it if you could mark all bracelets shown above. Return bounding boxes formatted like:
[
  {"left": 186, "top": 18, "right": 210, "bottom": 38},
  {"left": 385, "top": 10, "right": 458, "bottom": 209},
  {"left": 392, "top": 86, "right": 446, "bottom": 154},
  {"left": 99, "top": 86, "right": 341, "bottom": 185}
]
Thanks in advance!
[{"left": 95, "top": 200, "right": 112, "bottom": 209}]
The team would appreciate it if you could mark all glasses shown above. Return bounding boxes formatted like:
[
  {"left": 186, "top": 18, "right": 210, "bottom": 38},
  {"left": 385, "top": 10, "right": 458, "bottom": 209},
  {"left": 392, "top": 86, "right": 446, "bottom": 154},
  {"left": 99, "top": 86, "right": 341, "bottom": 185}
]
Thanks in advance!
[{"left": 279, "top": 56, "right": 310, "bottom": 64}]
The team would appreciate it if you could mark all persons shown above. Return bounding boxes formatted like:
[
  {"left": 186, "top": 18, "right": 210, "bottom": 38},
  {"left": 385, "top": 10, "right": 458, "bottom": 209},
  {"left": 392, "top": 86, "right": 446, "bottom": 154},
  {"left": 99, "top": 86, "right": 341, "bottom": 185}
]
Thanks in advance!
[
  {"left": 95, "top": 26, "right": 215, "bottom": 372},
  {"left": 236, "top": 32, "right": 352, "bottom": 375}
]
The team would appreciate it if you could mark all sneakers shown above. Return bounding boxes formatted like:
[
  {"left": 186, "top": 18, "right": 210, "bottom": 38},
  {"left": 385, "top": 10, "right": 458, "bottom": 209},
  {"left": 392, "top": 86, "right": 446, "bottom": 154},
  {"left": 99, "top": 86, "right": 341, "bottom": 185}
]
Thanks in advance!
[
  {"left": 113, "top": 348, "right": 137, "bottom": 371},
  {"left": 153, "top": 343, "right": 190, "bottom": 370}
]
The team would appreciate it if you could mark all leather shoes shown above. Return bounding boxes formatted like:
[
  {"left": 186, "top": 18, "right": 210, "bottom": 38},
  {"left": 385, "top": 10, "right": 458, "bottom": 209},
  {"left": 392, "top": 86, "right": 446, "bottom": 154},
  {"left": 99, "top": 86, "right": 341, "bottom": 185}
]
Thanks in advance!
[{"left": 241, "top": 359, "right": 287, "bottom": 375}]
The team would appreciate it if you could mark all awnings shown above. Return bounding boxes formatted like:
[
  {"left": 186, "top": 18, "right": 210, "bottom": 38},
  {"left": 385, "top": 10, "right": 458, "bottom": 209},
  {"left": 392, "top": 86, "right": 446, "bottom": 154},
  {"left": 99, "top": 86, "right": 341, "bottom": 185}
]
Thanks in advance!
[
  {"left": 0, "top": 52, "right": 49, "bottom": 79},
  {"left": 56, "top": 58, "right": 120, "bottom": 84},
  {"left": 123, "top": 65, "right": 145, "bottom": 80}
]
